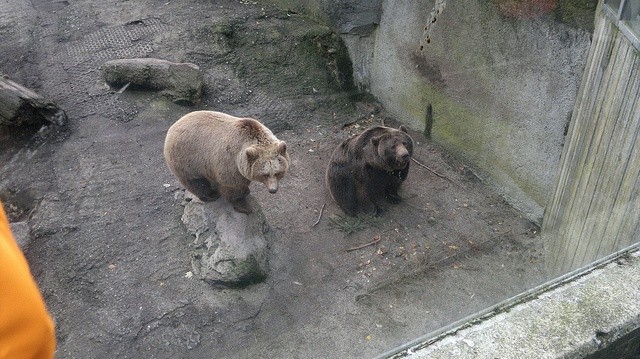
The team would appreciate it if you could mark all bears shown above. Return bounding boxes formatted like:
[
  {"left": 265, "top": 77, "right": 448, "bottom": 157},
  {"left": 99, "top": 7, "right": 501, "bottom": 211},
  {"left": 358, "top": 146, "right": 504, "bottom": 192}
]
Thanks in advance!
[
  {"left": 325, "top": 124, "right": 415, "bottom": 219},
  {"left": 163, "top": 111, "right": 291, "bottom": 215}
]
